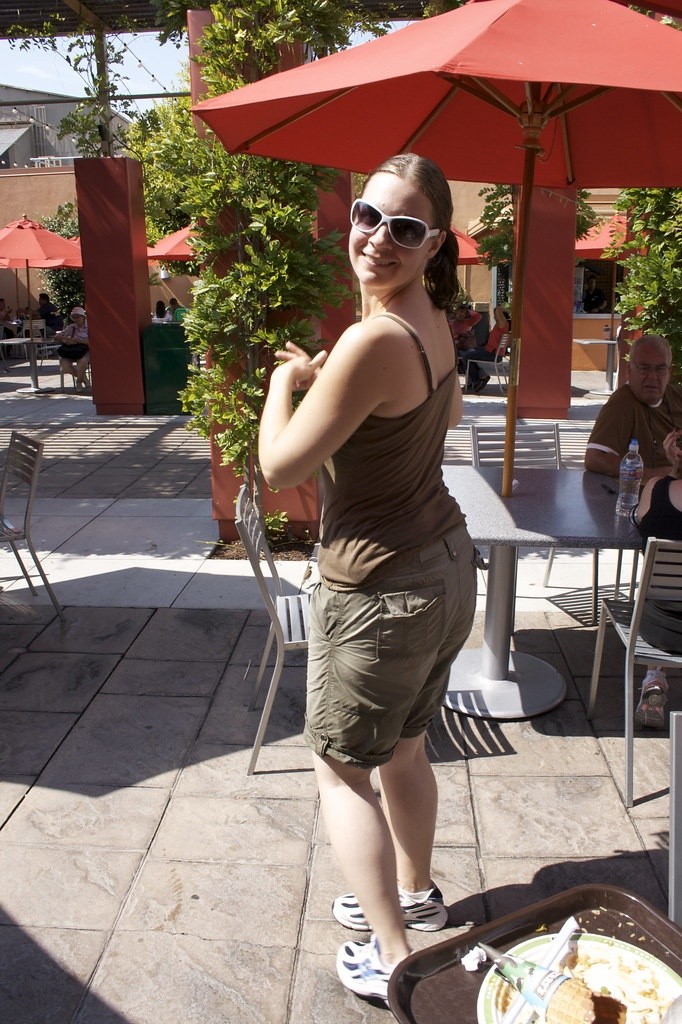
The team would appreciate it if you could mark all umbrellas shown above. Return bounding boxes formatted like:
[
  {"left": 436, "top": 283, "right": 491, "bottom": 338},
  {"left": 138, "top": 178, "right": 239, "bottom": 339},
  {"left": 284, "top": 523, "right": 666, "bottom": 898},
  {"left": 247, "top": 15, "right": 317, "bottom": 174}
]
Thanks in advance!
[
  {"left": 449, "top": 225, "right": 508, "bottom": 264},
  {"left": 67, "top": 224, "right": 212, "bottom": 263},
  {"left": 187, "top": 0, "right": 682, "bottom": 496},
  {"left": 0, "top": 211, "right": 83, "bottom": 339},
  {"left": 573, "top": 212, "right": 649, "bottom": 340}
]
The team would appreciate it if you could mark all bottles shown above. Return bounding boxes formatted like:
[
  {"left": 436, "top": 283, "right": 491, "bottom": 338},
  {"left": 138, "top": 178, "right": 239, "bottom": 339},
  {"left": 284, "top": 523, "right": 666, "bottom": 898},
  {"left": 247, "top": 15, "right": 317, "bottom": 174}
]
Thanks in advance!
[
  {"left": 603, "top": 324, "right": 611, "bottom": 340},
  {"left": 616, "top": 438, "right": 644, "bottom": 517}
]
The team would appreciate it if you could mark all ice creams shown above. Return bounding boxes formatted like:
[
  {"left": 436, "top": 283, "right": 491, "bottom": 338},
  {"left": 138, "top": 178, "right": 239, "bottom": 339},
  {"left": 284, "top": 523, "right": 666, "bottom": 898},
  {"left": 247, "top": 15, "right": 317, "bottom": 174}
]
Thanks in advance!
[{"left": 546, "top": 978, "right": 596, "bottom": 1024}]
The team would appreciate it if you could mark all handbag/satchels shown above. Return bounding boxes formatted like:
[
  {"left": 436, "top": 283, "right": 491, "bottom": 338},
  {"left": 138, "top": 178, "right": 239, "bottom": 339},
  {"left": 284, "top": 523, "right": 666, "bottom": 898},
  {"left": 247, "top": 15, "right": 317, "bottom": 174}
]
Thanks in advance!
[{"left": 57, "top": 343, "right": 88, "bottom": 359}]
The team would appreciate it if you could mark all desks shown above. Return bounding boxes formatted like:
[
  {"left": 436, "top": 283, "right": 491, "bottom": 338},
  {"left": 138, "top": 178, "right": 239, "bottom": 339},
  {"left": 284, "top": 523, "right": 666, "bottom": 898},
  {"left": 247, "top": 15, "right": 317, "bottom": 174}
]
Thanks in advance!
[
  {"left": 439, "top": 464, "right": 643, "bottom": 719},
  {"left": 572, "top": 339, "right": 618, "bottom": 395},
  {"left": 0, "top": 338, "right": 63, "bottom": 393}
]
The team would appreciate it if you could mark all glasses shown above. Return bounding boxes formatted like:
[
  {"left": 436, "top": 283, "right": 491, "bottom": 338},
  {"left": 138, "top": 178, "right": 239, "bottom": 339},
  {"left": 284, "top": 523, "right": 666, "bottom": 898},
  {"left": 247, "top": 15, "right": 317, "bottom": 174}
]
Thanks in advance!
[
  {"left": 350, "top": 199, "right": 439, "bottom": 249},
  {"left": 633, "top": 361, "right": 668, "bottom": 376}
]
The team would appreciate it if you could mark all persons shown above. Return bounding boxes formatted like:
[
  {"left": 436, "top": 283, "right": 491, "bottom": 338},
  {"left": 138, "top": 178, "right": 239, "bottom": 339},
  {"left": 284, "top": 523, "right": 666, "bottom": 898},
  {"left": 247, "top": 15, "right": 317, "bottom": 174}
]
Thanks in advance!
[
  {"left": 0, "top": 298, "right": 30, "bottom": 357},
  {"left": 585, "top": 334, "right": 682, "bottom": 486},
  {"left": 450, "top": 305, "right": 511, "bottom": 395},
  {"left": 32, "top": 294, "right": 63, "bottom": 357},
  {"left": 258, "top": 152, "right": 477, "bottom": 1011},
  {"left": 583, "top": 275, "right": 608, "bottom": 313},
  {"left": 54, "top": 306, "right": 92, "bottom": 394},
  {"left": 154, "top": 297, "right": 187, "bottom": 322},
  {"left": 634, "top": 431, "right": 682, "bottom": 730}
]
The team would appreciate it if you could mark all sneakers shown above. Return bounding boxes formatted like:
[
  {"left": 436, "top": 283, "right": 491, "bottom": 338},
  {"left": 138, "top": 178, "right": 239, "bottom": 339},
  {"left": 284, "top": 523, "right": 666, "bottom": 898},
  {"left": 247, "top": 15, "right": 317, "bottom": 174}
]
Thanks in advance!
[
  {"left": 336, "top": 934, "right": 416, "bottom": 1009},
  {"left": 635, "top": 670, "right": 669, "bottom": 727},
  {"left": 332, "top": 879, "right": 448, "bottom": 931}
]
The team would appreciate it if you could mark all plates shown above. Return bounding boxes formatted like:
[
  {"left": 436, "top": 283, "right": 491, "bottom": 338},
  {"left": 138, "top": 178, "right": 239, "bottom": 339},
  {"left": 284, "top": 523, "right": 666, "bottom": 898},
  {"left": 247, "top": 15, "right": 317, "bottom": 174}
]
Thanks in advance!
[{"left": 476, "top": 932, "right": 682, "bottom": 1024}]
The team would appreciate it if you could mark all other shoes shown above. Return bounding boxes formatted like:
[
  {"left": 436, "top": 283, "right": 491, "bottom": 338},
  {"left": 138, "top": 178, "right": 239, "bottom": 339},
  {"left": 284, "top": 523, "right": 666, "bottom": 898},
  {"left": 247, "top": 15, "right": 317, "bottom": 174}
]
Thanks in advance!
[
  {"left": 461, "top": 383, "right": 479, "bottom": 391},
  {"left": 475, "top": 375, "right": 490, "bottom": 392}
]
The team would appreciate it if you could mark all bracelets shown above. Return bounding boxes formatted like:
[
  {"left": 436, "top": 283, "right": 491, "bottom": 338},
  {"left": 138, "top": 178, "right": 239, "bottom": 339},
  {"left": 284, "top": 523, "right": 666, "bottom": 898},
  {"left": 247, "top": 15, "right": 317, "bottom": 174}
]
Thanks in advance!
[{"left": 598, "top": 305, "right": 602, "bottom": 310}]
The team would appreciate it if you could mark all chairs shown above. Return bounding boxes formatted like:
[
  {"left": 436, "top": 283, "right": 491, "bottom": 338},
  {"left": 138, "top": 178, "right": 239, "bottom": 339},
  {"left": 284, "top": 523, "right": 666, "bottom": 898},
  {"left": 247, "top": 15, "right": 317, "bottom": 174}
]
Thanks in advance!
[
  {"left": 470, "top": 423, "right": 681, "bottom": 809},
  {"left": 234, "top": 483, "right": 312, "bottom": 777},
  {"left": 0, "top": 431, "right": 70, "bottom": 629},
  {"left": 0, "top": 319, "right": 92, "bottom": 393},
  {"left": 464, "top": 333, "right": 508, "bottom": 395}
]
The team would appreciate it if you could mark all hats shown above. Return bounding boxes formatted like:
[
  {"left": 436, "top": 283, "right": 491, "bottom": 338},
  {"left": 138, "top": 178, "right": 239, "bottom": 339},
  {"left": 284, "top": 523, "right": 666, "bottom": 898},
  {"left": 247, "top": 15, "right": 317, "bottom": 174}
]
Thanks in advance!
[{"left": 70, "top": 307, "right": 86, "bottom": 321}]
